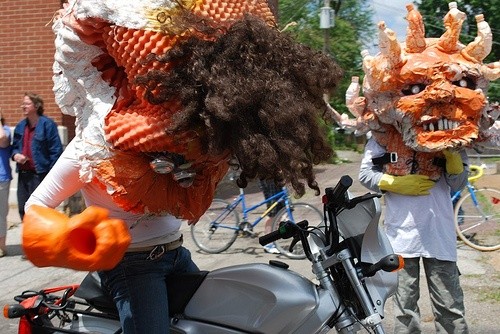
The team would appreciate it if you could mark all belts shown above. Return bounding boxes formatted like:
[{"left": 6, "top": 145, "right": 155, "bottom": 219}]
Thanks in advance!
[{"left": 126, "top": 237, "right": 183, "bottom": 260}]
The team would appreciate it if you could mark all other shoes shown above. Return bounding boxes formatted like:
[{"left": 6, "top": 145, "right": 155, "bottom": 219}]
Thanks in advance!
[
  {"left": 0, "top": 248, "right": 7, "bottom": 257},
  {"left": 264, "top": 242, "right": 280, "bottom": 254}
]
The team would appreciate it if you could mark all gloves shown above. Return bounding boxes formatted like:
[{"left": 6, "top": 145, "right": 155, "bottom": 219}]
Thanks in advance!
[
  {"left": 442, "top": 149, "right": 464, "bottom": 174},
  {"left": 377, "top": 173, "right": 434, "bottom": 195}
]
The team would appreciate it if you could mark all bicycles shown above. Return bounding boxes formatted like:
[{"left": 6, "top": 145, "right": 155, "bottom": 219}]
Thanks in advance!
[
  {"left": 449, "top": 163, "right": 500, "bottom": 253},
  {"left": 188, "top": 172, "right": 326, "bottom": 262}
]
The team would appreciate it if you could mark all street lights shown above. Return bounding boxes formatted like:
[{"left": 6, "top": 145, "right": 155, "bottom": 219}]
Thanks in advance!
[{"left": 318, "top": 5, "right": 336, "bottom": 52}]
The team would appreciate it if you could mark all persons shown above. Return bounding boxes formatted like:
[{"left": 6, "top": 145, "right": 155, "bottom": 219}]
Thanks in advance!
[
  {"left": 21, "top": 135, "right": 202, "bottom": 334},
  {"left": 258, "top": 165, "right": 289, "bottom": 254},
  {"left": 11, "top": 92, "right": 64, "bottom": 260},
  {"left": 0, "top": 112, "right": 13, "bottom": 257},
  {"left": 359, "top": 130, "right": 469, "bottom": 334}
]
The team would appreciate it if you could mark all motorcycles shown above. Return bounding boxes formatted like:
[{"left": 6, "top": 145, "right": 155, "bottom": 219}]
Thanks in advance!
[{"left": 4, "top": 170, "right": 408, "bottom": 334}]
